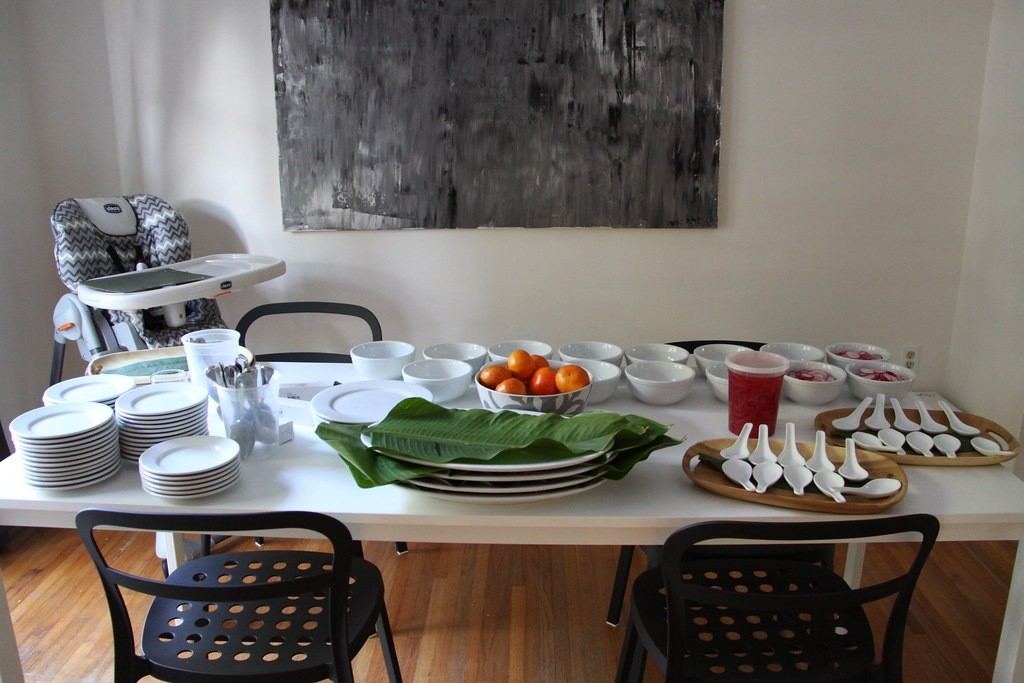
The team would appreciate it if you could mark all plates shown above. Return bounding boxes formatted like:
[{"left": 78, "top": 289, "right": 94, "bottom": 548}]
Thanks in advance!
[
  {"left": 309, "top": 378, "right": 612, "bottom": 499},
  {"left": 9, "top": 374, "right": 240, "bottom": 498}
]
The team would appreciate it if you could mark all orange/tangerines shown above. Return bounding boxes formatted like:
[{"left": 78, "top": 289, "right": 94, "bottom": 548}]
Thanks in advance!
[{"left": 480, "top": 350, "right": 590, "bottom": 396}]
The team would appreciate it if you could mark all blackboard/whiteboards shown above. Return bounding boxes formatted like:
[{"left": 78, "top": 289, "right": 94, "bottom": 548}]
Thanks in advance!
[{"left": 269, "top": 0, "right": 722, "bottom": 232}]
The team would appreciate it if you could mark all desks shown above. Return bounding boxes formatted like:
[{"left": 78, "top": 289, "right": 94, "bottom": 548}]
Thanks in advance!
[{"left": 0, "top": 363, "right": 1024, "bottom": 682}]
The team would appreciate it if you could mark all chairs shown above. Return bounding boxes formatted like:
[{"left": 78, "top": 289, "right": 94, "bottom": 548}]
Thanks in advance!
[
  {"left": 606, "top": 513, "right": 941, "bottom": 683},
  {"left": 233, "top": 302, "right": 409, "bottom": 556},
  {"left": 75, "top": 508, "right": 403, "bottom": 682},
  {"left": 50, "top": 194, "right": 265, "bottom": 577},
  {"left": 608, "top": 340, "right": 771, "bottom": 629}
]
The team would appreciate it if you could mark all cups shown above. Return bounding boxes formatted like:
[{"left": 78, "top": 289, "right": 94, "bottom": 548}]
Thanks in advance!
[
  {"left": 219, "top": 365, "right": 279, "bottom": 463},
  {"left": 150, "top": 368, "right": 187, "bottom": 385},
  {"left": 180, "top": 328, "right": 240, "bottom": 416},
  {"left": 723, "top": 350, "right": 787, "bottom": 440}
]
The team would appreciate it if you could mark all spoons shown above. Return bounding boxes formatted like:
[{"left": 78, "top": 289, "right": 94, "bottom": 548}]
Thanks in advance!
[
  {"left": 719, "top": 393, "right": 1016, "bottom": 504},
  {"left": 187, "top": 334, "right": 276, "bottom": 461}
]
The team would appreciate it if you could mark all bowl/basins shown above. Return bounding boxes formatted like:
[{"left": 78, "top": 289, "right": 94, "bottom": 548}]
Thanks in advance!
[
  {"left": 760, "top": 341, "right": 826, "bottom": 363},
  {"left": 846, "top": 360, "right": 916, "bottom": 405},
  {"left": 826, "top": 343, "right": 892, "bottom": 370},
  {"left": 558, "top": 341, "right": 624, "bottom": 366},
  {"left": 625, "top": 343, "right": 690, "bottom": 365},
  {"left": 350, "top": 340, "right": 418, "bottom": 378},
  {"left": 625, "top": 362, "right": 695, "bottom": 405},
  {"left": 401, "top": 359, "right": 474, "bottom": 402},
  {"left": 783, "top": 361, "right": 846, "bottom": 404},
  {"left": 564, "top": 358, "right": 622, "bottom": 404},
  {"left": 693, "top": 343, "right": 754, "bottom": 378},
  {"left": 423, "top": 342, "right": 488, "bottom": 377},
  {"left": 475, "top": 360, "right": 593, "bottom": 416},
  {"left": 487, "top": 340, "right": 553, "bottom": 362},
  {"left": 706, "top": 363, "right": 729, "bottom": 404}
]
